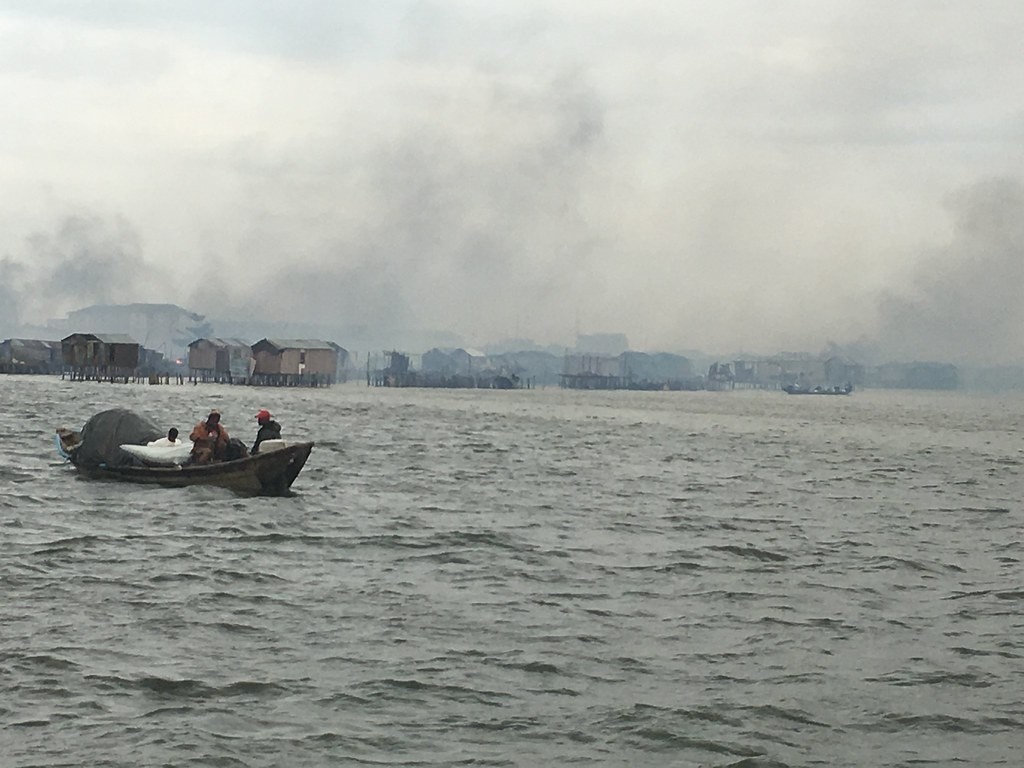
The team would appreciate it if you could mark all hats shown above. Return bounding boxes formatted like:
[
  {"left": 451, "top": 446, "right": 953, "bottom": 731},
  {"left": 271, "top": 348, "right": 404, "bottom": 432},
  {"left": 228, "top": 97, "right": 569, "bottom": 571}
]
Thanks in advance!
[{"left": 254, "top": 410, "right": 270, "bottom": 419}]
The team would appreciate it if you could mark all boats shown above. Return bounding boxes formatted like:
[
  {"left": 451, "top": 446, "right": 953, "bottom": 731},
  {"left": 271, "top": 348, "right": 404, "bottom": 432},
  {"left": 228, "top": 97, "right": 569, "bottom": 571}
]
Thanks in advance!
[
  {"left": 780, "top": 385, "right": 852, "bottom": 394},
  {"left": 56, "top": 408, "right": 315, "bottom": 497}
]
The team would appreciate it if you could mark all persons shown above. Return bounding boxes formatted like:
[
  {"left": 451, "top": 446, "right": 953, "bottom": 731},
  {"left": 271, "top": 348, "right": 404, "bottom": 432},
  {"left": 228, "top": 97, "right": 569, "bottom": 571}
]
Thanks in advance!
[
  {"left": 147, "top": 428, "right": 181, "bottom": 447},
  {"left": 250, "top": 410, "right": 281, "bottom": 456},
  {"left": 189, "top": 409, "right": 229, "bottom": 466}
]
